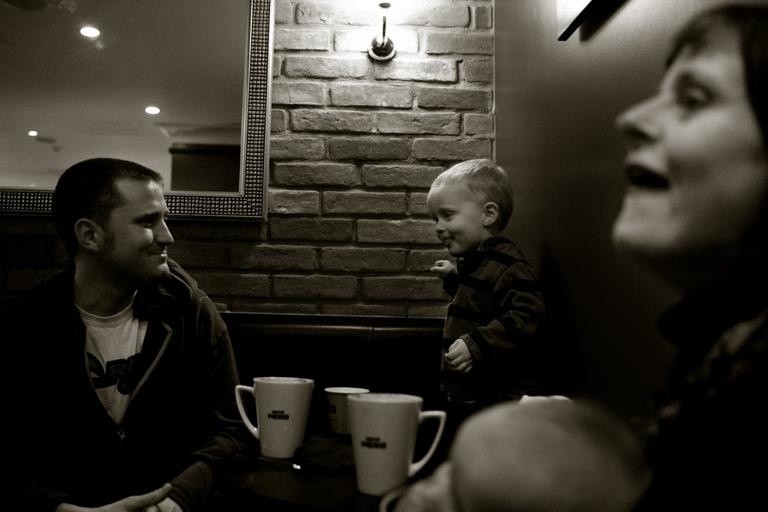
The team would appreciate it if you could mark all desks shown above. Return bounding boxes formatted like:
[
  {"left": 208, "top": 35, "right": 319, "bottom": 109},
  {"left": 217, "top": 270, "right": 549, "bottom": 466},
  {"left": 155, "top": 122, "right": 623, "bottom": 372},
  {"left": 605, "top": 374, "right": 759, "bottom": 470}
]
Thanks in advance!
[{"left": 214, "top": 417, "right": 446, "bottom": 510}]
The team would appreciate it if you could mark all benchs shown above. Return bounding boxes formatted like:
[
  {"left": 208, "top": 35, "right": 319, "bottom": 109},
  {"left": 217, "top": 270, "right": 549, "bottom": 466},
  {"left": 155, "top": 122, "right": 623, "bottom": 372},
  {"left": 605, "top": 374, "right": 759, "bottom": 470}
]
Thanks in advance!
[{"left": 213, "top": 309, "right": 448, "bottom": 434}]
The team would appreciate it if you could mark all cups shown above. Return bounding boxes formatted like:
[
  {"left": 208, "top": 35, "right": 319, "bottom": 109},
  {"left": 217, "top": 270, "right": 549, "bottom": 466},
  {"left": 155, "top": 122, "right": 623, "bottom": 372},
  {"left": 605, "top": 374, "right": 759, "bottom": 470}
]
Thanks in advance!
[
  {"left": 345, "top": 389, "right": 447, "bottom": 496},
  {"left": 232, "top": 373, "right": 313, "bottom": 461},
  {"left": 325, "top": 383, "right": 371, "bottom": 432}
]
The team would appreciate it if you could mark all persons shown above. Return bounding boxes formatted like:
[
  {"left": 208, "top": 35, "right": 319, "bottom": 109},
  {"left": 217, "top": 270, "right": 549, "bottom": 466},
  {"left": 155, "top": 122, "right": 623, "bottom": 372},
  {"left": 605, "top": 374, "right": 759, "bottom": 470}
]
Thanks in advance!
[
  {"left": 0, "top": 155, "right": 248, "bottom": 512},
  {"left": 401, "top": 2, "right": 766, "bottom": 512},
  {"left": 425, "top": 156, "right": 532, "bottom": 420}
]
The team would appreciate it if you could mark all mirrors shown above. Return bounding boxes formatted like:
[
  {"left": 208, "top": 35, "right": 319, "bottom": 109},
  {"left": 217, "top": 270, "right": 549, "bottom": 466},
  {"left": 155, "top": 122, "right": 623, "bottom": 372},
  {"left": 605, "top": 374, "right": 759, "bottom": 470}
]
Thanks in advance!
[{"left": 0, "top": 0, "right": 276, "bottom": 227}]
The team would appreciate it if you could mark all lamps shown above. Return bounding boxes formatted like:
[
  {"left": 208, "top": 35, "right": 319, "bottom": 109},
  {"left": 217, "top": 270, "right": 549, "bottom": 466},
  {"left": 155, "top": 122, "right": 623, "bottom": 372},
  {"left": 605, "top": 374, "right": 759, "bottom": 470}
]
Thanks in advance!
[{"left": 360, "top": 1, "right": 402, "bottom": 62}]
